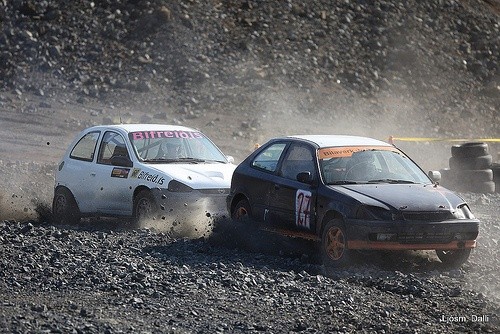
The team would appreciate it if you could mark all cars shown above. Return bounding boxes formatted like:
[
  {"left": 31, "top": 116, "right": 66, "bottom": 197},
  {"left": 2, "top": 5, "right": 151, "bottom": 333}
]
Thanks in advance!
[
  {"left": 51, "top": 123, "right": 238, "bottom": 230},
  {"left": 225, "top": 134, "right": 479, "bottom": 269}
]
